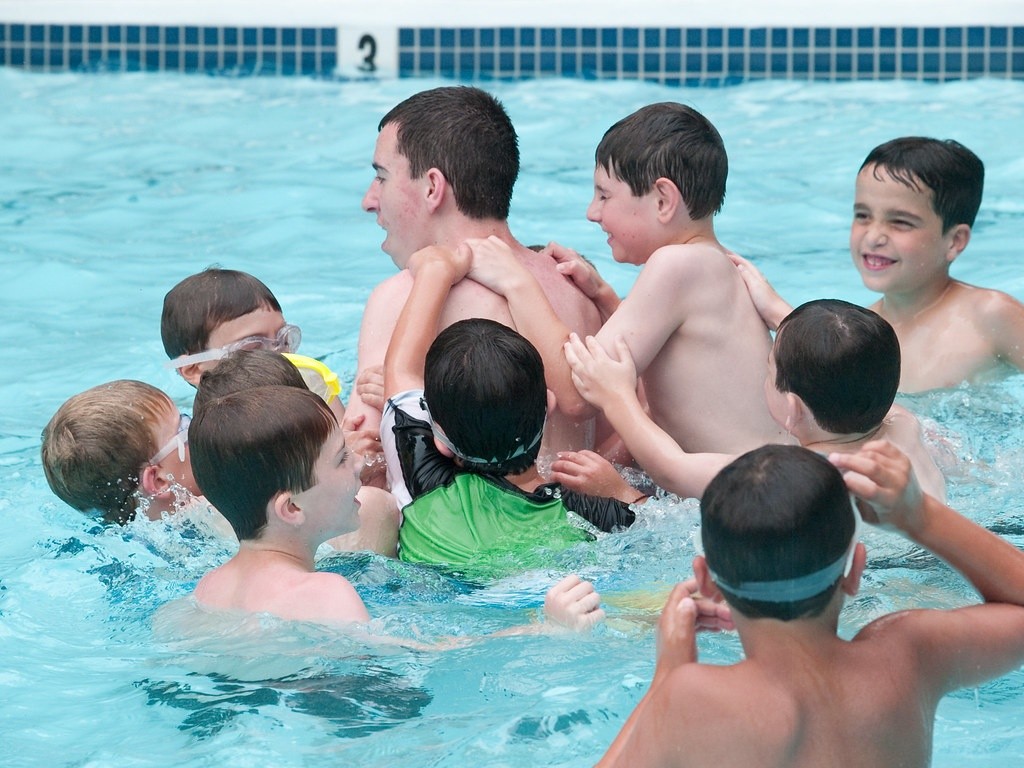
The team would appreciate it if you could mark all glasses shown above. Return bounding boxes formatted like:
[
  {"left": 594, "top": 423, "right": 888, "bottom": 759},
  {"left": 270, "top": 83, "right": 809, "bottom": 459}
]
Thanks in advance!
[
  {"left": 164, "top": 325, "right": 301, "bottom": 369},
  {"left": 140, "top": 414, "right": 192, "bottom": 473}
]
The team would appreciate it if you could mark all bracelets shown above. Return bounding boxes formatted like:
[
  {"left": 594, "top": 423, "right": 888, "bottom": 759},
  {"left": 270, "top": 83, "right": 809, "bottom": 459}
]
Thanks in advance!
[{"left": 628, "top": 493, "right": 653, "bottom": 506}]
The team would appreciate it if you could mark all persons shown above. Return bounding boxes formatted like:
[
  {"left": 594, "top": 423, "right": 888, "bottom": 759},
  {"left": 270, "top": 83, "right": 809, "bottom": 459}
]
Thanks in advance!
[
  {"left": 458, "top": 100, "right": 808, "bottom": 455},
  {"left": 156, "top": 265, "right": 389, "bottom": 500},
  {"left": 173, "top": 345, "right": 402, "bottom": 570},
  {"left": 146, "top": 380, "right": 605, "bottom": 657},
  {"left": 556, "top": 249, "right": 973, "bottom": 541},
  {"left": 39, "top": 380, "right": 214, "bottom": 560},
  {"left": 336, "top": 82, "right": 616, "bottom": 496},
  {"left": 595, "top": 440, "right": 1024, "bottom": 768},
  {"left": 849, "top": 134, "right": 1024, "bottom": 398},
  {"left": 375, "top": 237, "right": 661, "bottom": 572}
]
